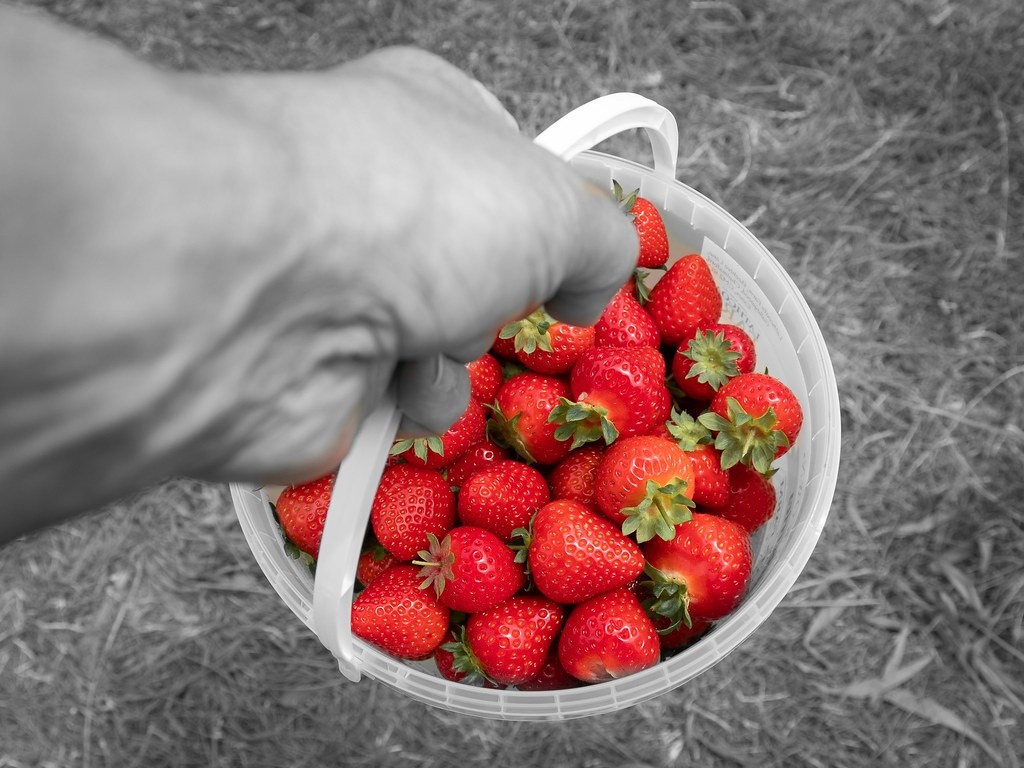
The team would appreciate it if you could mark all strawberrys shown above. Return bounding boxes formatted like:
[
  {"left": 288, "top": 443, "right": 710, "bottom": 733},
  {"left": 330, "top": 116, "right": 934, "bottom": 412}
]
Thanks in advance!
[{"left": 277, "top": 176, "right": 802, "bottom": 690}]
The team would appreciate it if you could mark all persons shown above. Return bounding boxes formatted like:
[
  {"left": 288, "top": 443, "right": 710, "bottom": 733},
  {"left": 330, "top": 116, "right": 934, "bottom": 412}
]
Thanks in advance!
[{"left": 0, "top": 2, "right": 639, "bottom": 547}]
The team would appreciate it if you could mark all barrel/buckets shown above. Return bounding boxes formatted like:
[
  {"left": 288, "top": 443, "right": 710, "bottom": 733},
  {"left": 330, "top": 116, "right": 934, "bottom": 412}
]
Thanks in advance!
[{"left": 227, "top": 91, "right": 840, "bottom": 723}]
